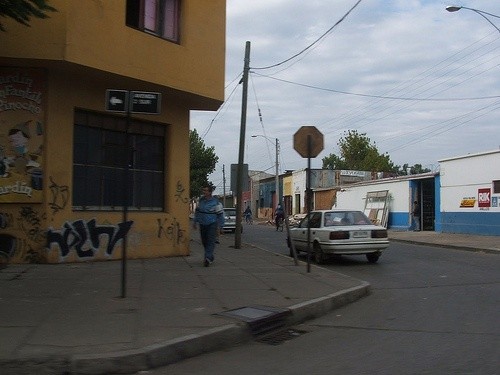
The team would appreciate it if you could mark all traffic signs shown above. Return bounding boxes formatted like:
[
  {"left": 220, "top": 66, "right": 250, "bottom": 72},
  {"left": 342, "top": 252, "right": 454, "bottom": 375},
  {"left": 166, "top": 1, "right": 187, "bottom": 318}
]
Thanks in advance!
[{"left": 104, "top": 89, "right": 162, "bottom": 115}]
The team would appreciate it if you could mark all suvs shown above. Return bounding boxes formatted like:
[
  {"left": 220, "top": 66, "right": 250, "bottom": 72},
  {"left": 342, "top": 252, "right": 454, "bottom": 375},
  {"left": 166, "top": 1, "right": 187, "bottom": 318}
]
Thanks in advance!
[{"left": 222, "top": 207, "right": 243, "bottom": 234}]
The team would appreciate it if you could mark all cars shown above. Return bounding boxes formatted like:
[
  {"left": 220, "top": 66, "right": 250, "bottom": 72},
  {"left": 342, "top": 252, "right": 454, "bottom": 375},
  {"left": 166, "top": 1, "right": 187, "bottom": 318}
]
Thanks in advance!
[{"left": 285, "top": 209, "right": 390, "bottom": 265}]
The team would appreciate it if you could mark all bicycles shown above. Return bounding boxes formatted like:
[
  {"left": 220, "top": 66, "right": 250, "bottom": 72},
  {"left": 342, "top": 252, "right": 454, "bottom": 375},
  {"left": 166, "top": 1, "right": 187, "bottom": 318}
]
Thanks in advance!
[{"left": 274, "top": 214, "right": 283, "bottom": 232}]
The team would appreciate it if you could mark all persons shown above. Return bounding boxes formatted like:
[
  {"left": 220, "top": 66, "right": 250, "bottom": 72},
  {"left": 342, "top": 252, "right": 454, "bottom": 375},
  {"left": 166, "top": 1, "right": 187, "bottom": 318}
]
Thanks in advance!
[
  {"left": 411, "top": 201, "right": 421, "bottom": 232},
  {"left": 275, "top": 203, "right": 284, "bottom": 228},
  {"left": 245, "top": 206, "right": 252, "bottom": 224},
  {"left": 193, "top": 185, "right": 225, "bottom": 267}
]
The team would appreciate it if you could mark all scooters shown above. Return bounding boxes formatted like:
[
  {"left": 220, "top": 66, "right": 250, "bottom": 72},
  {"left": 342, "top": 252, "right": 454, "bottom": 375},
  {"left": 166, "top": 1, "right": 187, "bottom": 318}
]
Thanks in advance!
[{"left": 245, "top": 213, "right": 252, "bottom": 224}]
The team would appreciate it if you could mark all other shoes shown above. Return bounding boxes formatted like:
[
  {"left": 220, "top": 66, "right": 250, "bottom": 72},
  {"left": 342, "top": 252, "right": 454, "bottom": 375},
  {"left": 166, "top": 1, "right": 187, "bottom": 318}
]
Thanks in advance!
[{"left": 205, "top": 257, "right": 211, "bottom": 267}]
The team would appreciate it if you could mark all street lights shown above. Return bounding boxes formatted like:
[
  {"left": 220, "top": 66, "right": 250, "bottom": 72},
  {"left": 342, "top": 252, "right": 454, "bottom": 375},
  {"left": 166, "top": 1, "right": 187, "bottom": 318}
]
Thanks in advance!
[{"left": 251, "top": 135, "right": 279, "bottom": 209}]
